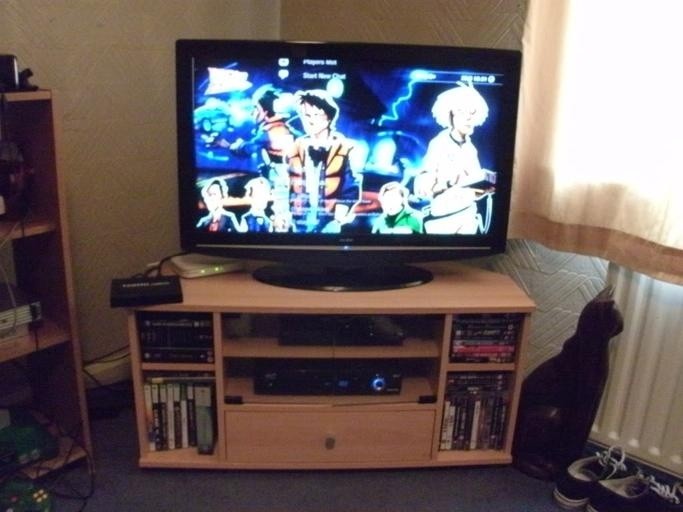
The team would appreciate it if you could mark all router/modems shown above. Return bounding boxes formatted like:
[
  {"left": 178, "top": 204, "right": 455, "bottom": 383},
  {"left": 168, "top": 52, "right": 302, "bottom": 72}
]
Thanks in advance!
[
  {"left": 172, "top": 254, "right": 238, "bottom": 278},
  {"left": 110, "top": 276, "right": 182, "bottom": 308}
]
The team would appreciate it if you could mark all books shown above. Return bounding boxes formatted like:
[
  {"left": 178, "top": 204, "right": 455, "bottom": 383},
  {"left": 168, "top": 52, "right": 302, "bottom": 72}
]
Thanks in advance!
[
  {"left": 438, "top": 314, "right": 522, "bottom": 451},
  {"left": 142, "top": 381, "right": 216, "bottom": 456}
]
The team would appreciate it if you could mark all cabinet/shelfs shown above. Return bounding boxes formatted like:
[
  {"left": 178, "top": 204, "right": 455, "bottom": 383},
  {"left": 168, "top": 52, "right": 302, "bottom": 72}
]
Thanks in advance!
[
  {"left": 0, "top": 89, "right": 95, "bottom": 482},
  {"left": 126, "top": 262, "right": 537, "bottom": 472}
]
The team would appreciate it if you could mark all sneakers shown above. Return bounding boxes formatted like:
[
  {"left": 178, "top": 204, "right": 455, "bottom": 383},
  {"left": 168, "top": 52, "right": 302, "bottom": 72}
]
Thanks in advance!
[{"left": 554, "top": 444, "right": 683, "bottom": 496}]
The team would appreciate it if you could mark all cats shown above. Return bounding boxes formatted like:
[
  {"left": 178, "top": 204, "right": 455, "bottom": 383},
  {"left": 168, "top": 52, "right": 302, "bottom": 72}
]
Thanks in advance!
[{"left": 511, "top": 283, "right": 625, "bottom": 481}]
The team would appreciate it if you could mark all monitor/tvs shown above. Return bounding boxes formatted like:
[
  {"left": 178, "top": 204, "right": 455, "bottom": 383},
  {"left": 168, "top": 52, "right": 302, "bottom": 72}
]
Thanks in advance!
[{"left": 175, "top": 38, "right": 523, "bottom": 291}]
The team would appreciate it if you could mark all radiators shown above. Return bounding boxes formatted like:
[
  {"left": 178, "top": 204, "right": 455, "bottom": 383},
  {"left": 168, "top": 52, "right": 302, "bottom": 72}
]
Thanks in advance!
[{"left": 586, "top": 261, "right": 683, "bottom": 481}]
